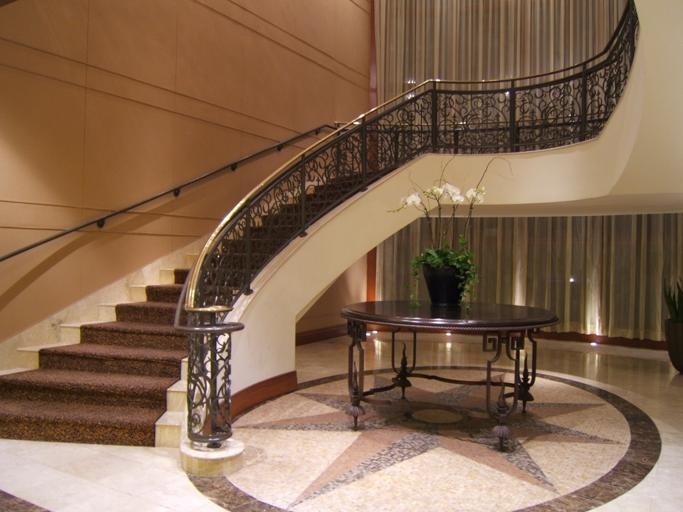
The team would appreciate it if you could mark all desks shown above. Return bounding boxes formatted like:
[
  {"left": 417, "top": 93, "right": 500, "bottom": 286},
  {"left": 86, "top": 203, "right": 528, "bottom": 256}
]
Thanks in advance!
[{"left": 340, "top": 300, "right": 559, "bottom": 452}]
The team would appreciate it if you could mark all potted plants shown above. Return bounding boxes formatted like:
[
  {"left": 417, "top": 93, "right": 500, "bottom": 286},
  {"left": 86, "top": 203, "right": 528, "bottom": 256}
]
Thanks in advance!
[{"left": 661, "top": 275, "right": 683, "bottom": 373}]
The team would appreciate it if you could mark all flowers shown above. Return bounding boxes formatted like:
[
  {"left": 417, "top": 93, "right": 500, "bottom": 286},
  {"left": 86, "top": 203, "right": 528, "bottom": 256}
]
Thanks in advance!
[{"left": 387, "top": 153, "right": 511, "bottom": 313}]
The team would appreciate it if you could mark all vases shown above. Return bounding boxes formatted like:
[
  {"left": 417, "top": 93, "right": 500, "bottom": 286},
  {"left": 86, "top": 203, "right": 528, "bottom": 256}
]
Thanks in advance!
[{"left": 421, "top": 262, "right": 466, "bottom": 308}]
